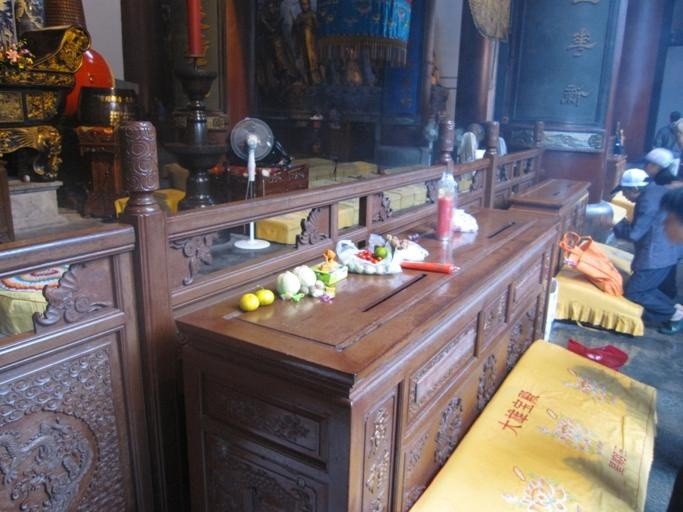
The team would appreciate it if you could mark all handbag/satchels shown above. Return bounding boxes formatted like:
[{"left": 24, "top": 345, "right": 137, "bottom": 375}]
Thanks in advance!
[{"left": 559, "top": 231, "right": 624, "bottom": 296}]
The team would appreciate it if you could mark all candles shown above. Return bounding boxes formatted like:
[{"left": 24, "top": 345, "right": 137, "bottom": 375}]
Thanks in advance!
[
  {"left": 436, "top": 193, "right": 451, "bottom": 241},
  {"left": 399, "top": 261, "right": 460, "bottom": 275}
]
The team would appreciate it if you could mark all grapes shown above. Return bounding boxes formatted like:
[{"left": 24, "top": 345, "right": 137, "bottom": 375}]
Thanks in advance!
[{"left": 356, "top": 250, "right": 382, "bottom": 264}]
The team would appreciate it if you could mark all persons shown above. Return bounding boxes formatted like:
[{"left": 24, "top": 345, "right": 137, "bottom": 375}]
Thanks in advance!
[
  {"left": 669, "top": 117, "right": 682, "bottom": 159},
  {"left": 642, "top": 146, "right": 683, "bottom": 186},
  {"left": 657, "top": 184, "right": 683, "bottom": 247},
  {"left": 604, "top": 166, "right": 683, "bottom": 336},
  {"left": 653, "top": 110, "right": 682, "bottom": 150}
]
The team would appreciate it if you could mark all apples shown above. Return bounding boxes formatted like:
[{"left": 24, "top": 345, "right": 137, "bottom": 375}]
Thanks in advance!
[{"left": 375, "top": 247, "right": 387, "bottom": 258}]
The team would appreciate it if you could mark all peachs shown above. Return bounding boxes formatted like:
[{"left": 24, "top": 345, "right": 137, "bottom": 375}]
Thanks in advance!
[{"left": 275, "top": 264, "right": 317, "bottom": 297}]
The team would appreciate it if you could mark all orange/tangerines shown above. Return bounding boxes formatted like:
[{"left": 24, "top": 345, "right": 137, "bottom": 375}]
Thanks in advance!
[{"left": 238, "top": 288, "right": 276, "bottom": 312}]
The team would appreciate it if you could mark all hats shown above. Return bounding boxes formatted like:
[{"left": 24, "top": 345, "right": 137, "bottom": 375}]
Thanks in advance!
[{"left": 611, "top": 168, "right": 650, "bottom": 194}]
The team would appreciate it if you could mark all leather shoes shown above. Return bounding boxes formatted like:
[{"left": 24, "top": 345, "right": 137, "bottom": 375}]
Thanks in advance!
[{"left": 659, "top": 320, "right": 683, "bottom": 335}]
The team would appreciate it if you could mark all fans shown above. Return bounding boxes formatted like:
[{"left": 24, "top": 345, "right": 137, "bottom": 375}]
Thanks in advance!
[{"left": 229, "top": 118, "right": 274, "bottom": 250}]
